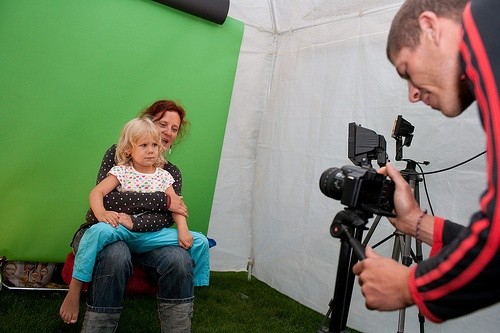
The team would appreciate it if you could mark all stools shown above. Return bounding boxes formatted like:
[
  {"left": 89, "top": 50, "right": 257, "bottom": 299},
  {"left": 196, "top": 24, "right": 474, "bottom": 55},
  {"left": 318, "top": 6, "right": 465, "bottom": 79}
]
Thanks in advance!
[{"left": 61, "top": 252, "right": 161, "bottom": 299}]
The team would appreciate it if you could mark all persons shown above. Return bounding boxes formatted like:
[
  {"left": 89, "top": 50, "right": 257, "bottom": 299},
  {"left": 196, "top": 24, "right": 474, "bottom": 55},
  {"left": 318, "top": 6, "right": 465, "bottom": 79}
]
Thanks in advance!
[
  {"left": 353, "top": 0, "right": 500, "bottom": 325},
  {"left": 60, "top": 118, "right": 210, "bottom": 324},
  {"left": 70, "top": 99, "right": 191, "bottom": 333}
]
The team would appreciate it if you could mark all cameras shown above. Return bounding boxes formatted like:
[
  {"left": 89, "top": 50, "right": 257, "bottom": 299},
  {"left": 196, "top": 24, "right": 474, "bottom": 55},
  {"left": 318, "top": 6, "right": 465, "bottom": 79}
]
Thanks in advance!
[{"left": 319, "top": 122, "right": 397, "bottom": 217}]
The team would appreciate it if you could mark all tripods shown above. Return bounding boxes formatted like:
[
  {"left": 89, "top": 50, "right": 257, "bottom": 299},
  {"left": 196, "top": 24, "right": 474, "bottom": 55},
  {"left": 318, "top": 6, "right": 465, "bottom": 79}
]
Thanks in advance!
[{"left": 322, "top": 159, "right": 436, "bottom": 333}]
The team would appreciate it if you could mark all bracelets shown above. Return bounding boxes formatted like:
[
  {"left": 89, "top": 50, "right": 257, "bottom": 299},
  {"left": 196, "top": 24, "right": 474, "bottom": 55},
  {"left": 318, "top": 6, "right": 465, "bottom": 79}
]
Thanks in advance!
[
  {"left": 415, "top": 209, "right": 428, "bottom": 244},
  {"left": 166, "top": 194, "right": 172, "bottom": 209}
]
